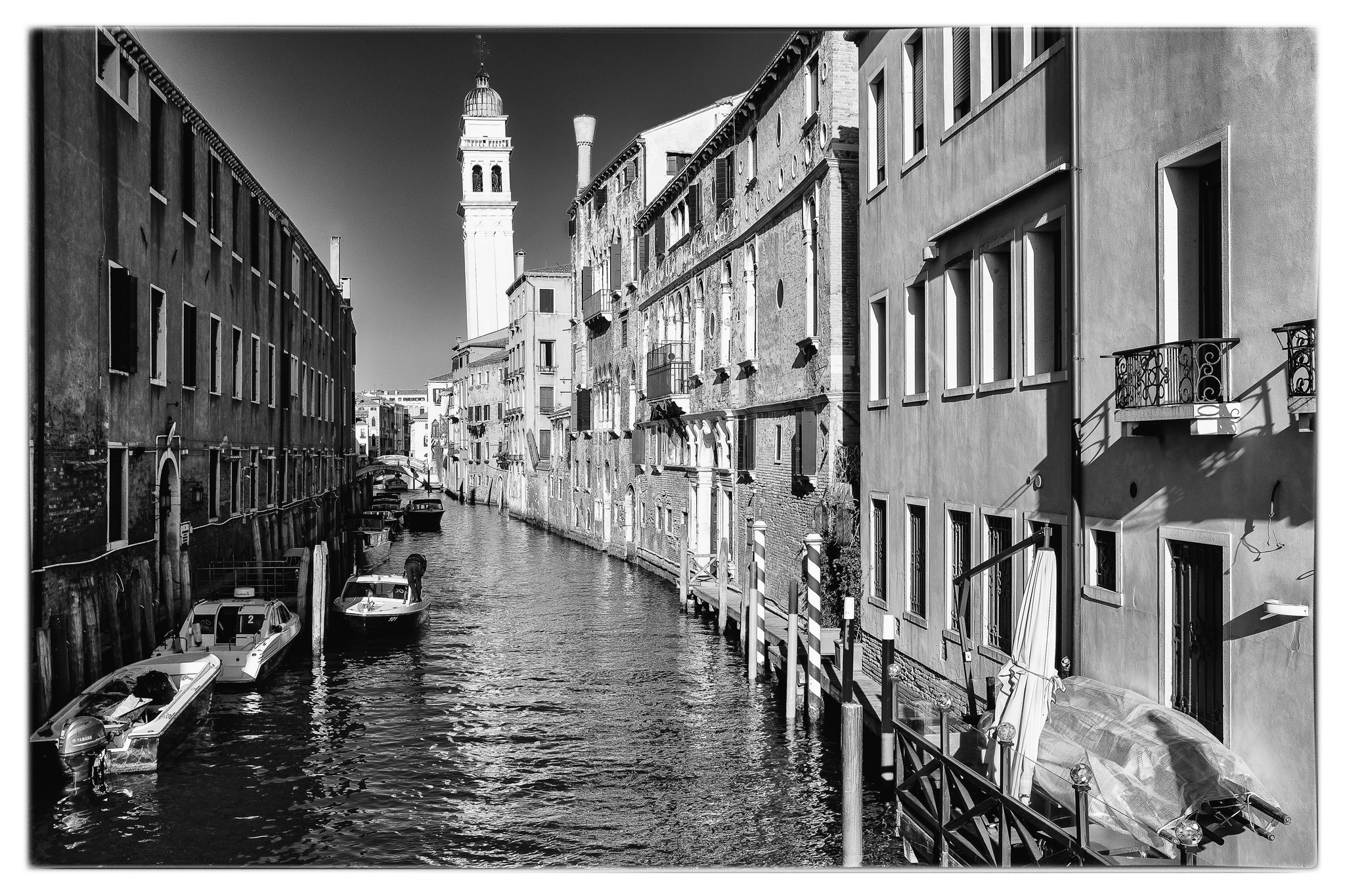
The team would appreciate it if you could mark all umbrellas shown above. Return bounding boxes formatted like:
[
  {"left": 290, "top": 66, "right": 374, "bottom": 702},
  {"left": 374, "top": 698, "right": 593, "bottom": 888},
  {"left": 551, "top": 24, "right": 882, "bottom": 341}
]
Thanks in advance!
[{"left": 983, "top": 548, "right": 1064, "bottom": 811}]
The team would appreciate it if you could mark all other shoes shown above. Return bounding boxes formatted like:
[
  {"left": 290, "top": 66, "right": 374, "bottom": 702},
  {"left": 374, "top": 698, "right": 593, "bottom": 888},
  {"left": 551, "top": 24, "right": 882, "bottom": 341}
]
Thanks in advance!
[
  {"left": 413, "top": 597, "right": 419, "bottom": 603},
  {"left": 419, "top": 597, "right": 422, "bottom": 602}
]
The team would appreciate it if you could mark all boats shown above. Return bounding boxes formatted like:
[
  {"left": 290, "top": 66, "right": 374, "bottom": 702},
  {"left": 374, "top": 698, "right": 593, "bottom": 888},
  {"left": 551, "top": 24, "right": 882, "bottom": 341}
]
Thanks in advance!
[
  {"left": 332, "top": 568, "right": 432, "bottom": 634},
  {"left": 344, "top": 492, "right": 403, "bottom": 571},
  {"left": 374, "top": 476, "right": 384, "bottom": 483},
  {"left": 384, "top": 478, "right": 408, "bottom": 491},
  {"left": 30, "top": 652, "right": 223, "bottom": 776},
  {"left": 407, "top": 499, "right": 446, "bottom": 525},
  {"left": 149, "top": 587, "right": 302, "bottom": 689}
]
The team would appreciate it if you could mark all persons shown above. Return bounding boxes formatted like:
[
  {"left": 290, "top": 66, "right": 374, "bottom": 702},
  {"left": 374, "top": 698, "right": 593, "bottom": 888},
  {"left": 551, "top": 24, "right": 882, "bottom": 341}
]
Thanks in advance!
[{"left": 404, "top": 554, "right": 427, "bottom": 603}]
